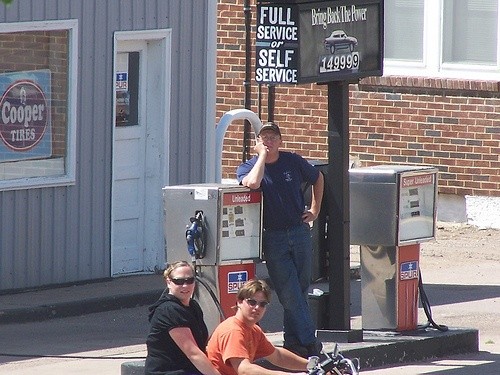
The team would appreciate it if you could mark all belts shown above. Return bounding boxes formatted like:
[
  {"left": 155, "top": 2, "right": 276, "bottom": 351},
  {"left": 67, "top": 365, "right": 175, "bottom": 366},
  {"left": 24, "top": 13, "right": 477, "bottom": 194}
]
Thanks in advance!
[{"left": 263, "top": 220, "right": 305, "bottom": 230}]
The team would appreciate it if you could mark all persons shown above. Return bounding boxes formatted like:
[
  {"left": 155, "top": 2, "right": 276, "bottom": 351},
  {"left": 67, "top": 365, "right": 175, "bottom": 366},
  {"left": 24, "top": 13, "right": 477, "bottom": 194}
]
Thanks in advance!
[
  {"left": 236, "top": 122, "right": 324, "bottom": 359},
  {"left": 145, "top": 261, "right": 222, "bottom": 375},
  {"left": 207, "top": 279, "right": 309, "bottom": 375}
]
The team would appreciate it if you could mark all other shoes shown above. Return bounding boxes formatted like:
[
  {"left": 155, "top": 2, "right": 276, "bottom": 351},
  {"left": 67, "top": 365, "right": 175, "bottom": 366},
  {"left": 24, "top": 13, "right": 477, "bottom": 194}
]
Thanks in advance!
[{"left": 283, "top": 338, "right": 324, "bottom": 361}]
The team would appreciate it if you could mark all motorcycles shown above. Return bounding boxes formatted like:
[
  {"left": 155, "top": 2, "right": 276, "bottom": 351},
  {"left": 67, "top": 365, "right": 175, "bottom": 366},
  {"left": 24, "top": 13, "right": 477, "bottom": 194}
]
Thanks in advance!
[{"left": 305, "top": 343, "right": 362, "bottom": 375}]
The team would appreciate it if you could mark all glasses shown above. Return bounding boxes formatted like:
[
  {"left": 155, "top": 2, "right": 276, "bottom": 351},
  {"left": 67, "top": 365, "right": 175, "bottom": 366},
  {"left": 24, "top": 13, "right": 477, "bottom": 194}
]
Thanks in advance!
[
  {"left": 169, "top": 275, "right": 195, "bottom": 286},
  {"left": 244, "top": 297, "right": 268, "bottom": 308},
  {"left": 259, "top": 134, "right": 278, "bottom": 141}
]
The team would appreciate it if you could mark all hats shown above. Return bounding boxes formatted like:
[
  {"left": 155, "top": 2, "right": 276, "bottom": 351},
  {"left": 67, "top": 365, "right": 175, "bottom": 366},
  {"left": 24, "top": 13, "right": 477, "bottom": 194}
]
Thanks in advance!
[{"left": 256, "top": 122, "right": 282, "bottom": 138}]
